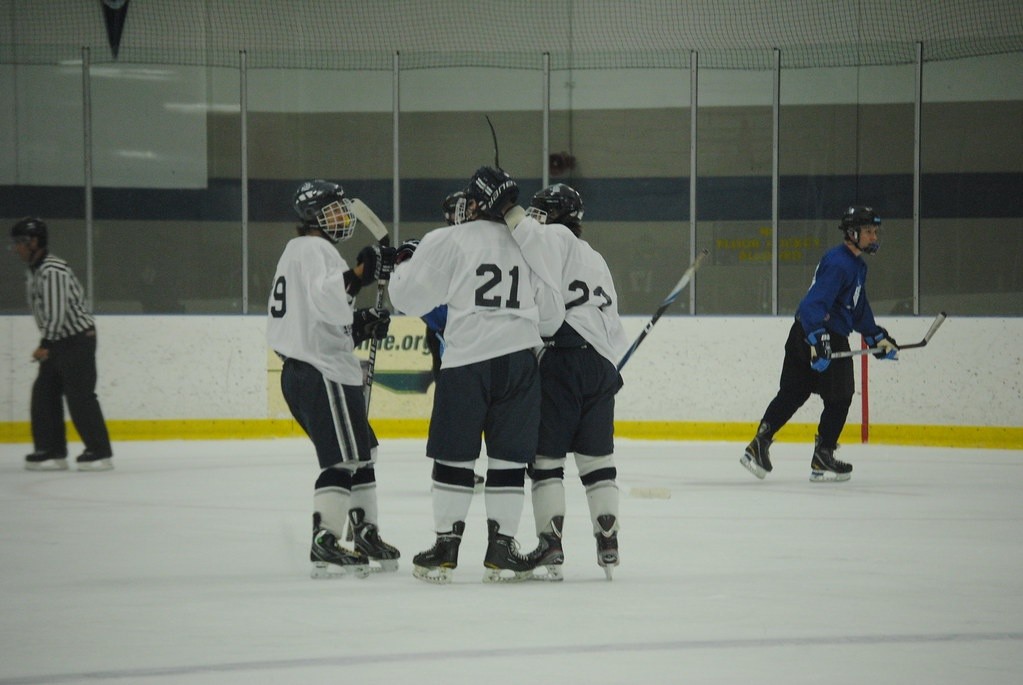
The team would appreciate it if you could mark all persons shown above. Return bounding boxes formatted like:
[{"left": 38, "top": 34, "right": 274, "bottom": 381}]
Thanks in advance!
[
  {"left": 386, "top": 164, "right": 626, "bottom": 573},
  {"left": 264, "top": 177, "right": 401, "bottom": 568},
  {"left": 743, "top": 203, "right": 900, "bottom": 473},
  {"left": 10, "top": 217, "right": 113, "bottom": 463}
]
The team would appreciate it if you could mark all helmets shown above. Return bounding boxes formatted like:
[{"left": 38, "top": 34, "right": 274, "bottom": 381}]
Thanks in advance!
[
  {"left": 455, "top": 180, "right": 506, "bottom": 224},
  {"left": 526, "top": 182, "right": 583, "bottom": 227},
  {"left": 293, "top": 180, "right": 354, "bottom": 243},
  {"left": 11, "top": 218, "right": 48, "bottom": 247},
  {"left": 838, "top": 206, "right": 881, "bottom": 233},
  {"left": 443, "top": 191, "right": 467, "bottom": 223}
]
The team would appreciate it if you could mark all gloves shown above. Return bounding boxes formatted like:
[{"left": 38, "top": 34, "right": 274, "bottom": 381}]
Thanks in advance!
[
  {"left": 864, "top": 327, "right": 900, "bottom": 362},
  {"left": 352, "top": 305, "right": 392, "bottom": 341},
  {"left": 472, "top": 166, "right": 519, "bottom": 215},
  {"left": 356, "top": 245, "right": 394, "bottom": 285},
  {"left": 806, "top": 329, "right": 831, "bottom": 373},
  {"left": 394, "top": 237, "right": 421, "bottom": 260}
]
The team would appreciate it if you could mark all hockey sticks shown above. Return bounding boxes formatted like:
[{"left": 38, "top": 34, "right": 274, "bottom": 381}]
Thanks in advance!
[
  {"left": 615, "top": 247, "right": 710, "bottom": 373},
  {"left": 813, "top": 310, "right": 948, "bottom": 361},
  {"left": 343, "top": 194, "right": 395, "bottom": 541}
]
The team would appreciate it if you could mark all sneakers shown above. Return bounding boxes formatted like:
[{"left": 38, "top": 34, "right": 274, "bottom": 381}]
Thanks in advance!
[
  {"left": 527, "top": 517, "right": 564, "bottom": 582},
  {"left": 310, "top": 512, "right": 369, "bottom": 579},
  {"left": 412, "top": 521, "right": 465, "bottom": 584},
  {"left": 346, "top": 507, "right": 400, "bottom": 571},
  {"left": 810, "top": 434, "right": 852, "bottom": 484},
  {"left": 25, "top": 448, "right": 68, "bottom": 471},
  {"left": 77, "top": 443, "right": 112, "bottom": 472},
  {"left": 483, "top": 519, "right": 532, "bottom": 583},
  {"left": 740, "top": 421, "right": 776, "bottom": 480},
  {"left": 592, "top": 514, "right": 621, "bottom": 580}
]
